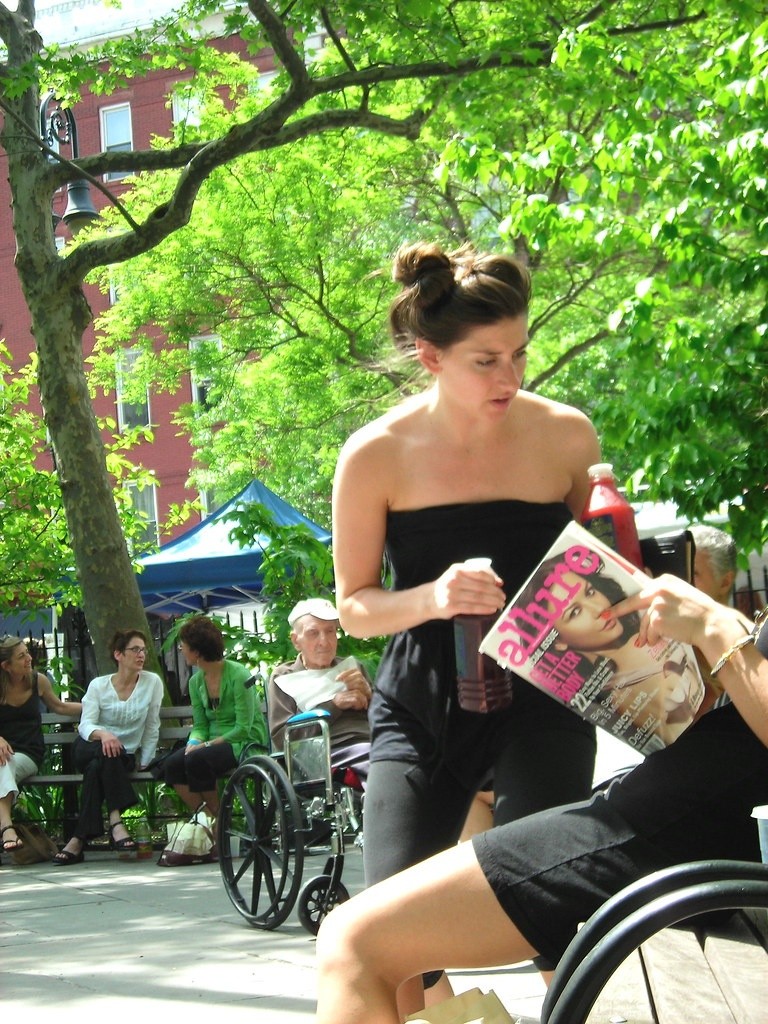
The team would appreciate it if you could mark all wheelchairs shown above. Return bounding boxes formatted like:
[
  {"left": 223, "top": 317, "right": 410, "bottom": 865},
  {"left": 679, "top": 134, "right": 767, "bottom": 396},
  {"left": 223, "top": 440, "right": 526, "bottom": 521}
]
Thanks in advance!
[{"left": 217, "top": 674, "right": 368, "bottom": 936}]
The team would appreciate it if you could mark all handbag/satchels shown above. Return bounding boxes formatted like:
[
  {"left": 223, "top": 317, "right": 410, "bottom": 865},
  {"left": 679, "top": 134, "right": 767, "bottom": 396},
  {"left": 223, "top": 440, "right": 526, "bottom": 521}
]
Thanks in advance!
[{"left": 156, "top": 801, "right": 218, "bottom": 867}]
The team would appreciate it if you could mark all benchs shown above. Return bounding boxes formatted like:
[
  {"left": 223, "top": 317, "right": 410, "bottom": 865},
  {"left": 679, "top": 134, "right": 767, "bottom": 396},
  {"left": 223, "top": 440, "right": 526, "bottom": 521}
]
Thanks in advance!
[{"left": 8, "top": 706, "right": 272, "bottom": 857}]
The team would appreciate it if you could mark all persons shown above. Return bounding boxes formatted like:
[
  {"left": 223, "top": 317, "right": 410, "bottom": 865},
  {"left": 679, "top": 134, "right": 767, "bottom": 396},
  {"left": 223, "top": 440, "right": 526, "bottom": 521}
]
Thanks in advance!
[
  {"left": 52, "top": 629, "right": 165, "bottom": 863},
  {"left": 332, "top": 240, "right": 602, "bottom": 1008},
  {"left": 315, "top": 526, "right": 768, "bottom": 1024},
  {"left": 510, "top": 552, "right": 722, "bottom": 744},
  {"left": 0, "top": 636, "right": 82, "bottom": 852},
  {"left": 268, "top": 598, "right": 371, "bottom": 776},
  {"left": 163, "top": 614, "right": 270, "bottom": 862}
]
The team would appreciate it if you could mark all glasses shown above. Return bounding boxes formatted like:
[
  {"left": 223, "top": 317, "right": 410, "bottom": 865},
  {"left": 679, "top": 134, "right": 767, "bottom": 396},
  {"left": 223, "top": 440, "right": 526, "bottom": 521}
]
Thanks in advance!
[
  {"left": 178, "top": 645, "right": 192, "bottom": 652},
  {"left": 122, "top": 647, "right": 149, "bottom": 656}
]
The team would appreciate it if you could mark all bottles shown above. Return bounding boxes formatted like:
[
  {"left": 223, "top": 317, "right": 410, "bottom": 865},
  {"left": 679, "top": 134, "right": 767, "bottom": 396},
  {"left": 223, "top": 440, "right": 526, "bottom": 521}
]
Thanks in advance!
[
  {"left": 136, "top": 818, "right": 151, "bottom": 858},
  {"left": 455, "top": 558, "right": 512, "bottom": 712},
  {"left": 580, "top": 462, "right": 646, "bottom": 574}
]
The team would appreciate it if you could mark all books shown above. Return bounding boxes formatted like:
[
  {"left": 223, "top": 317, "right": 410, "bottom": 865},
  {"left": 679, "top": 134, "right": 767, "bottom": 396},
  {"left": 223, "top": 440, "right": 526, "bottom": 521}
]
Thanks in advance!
[{"left": 477, "top": 521, "right": 755, "bottom": 756}]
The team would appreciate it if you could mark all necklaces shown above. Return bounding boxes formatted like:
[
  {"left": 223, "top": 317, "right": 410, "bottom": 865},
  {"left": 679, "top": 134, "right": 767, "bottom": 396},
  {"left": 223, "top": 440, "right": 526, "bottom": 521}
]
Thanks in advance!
[{"left": 205, "top": 675, "right": 218, "bottom": 710}]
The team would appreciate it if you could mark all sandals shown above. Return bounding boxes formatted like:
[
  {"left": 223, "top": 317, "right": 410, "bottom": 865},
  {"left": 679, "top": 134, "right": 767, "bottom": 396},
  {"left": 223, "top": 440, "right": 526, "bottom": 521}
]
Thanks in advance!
[
  {"left": 0, "top": 825, "right": 23, "bottom": 853},
  {"left": 53, "top": 850, "right": 84, "bottom": 863},
  {"left": 109, "top": 821, "right": 137, "bottom": 850}
]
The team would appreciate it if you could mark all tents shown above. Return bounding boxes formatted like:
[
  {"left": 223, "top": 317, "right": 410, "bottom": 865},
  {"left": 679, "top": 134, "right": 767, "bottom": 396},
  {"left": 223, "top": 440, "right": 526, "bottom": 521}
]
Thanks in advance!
[{"left": 51, "top": 478, "right": 333, "bottom": 668}]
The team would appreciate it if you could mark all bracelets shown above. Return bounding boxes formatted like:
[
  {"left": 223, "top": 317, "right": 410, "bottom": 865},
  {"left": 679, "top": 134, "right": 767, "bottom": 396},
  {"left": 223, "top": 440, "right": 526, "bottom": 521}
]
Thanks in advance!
[
  {"left": 709, "top": 632, "right": 760, "bottom": 677},
  {"left": 204, "top": 740, "right": 210, "bottom": 747}
]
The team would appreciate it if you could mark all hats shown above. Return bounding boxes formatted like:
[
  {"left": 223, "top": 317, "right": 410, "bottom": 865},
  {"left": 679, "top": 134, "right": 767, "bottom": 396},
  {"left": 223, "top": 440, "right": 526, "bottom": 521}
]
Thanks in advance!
[{"left": 287, "top": 598, "right": 340, "bottom": 627}]
